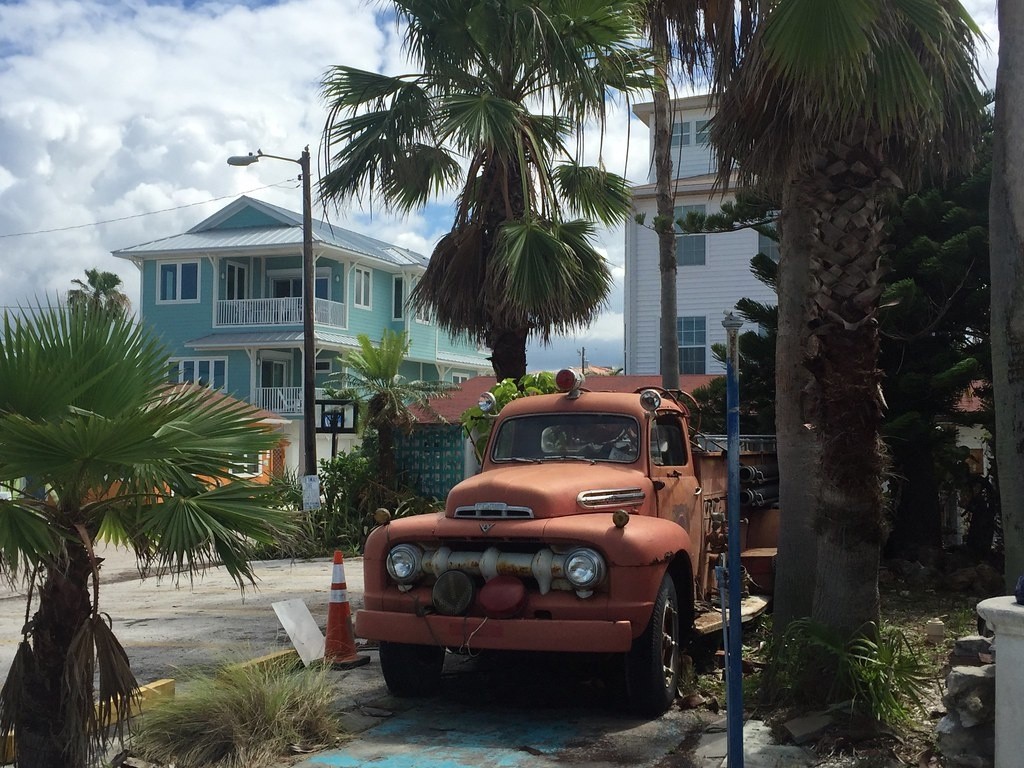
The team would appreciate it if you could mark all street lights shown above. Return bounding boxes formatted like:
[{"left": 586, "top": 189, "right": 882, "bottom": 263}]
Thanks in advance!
[{"left": 227, "top": 146, "right": 318, "bottom": 477}]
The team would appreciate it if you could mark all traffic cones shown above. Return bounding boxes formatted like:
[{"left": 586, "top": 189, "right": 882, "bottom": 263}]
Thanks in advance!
[{"left": 309, "top": 549, "right": 371, "bottom": 670}]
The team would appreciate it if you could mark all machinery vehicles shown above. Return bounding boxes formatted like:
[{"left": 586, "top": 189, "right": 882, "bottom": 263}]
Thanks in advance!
[{"left": 349, "top": 369, "right": 786, "bottom": 722}]
